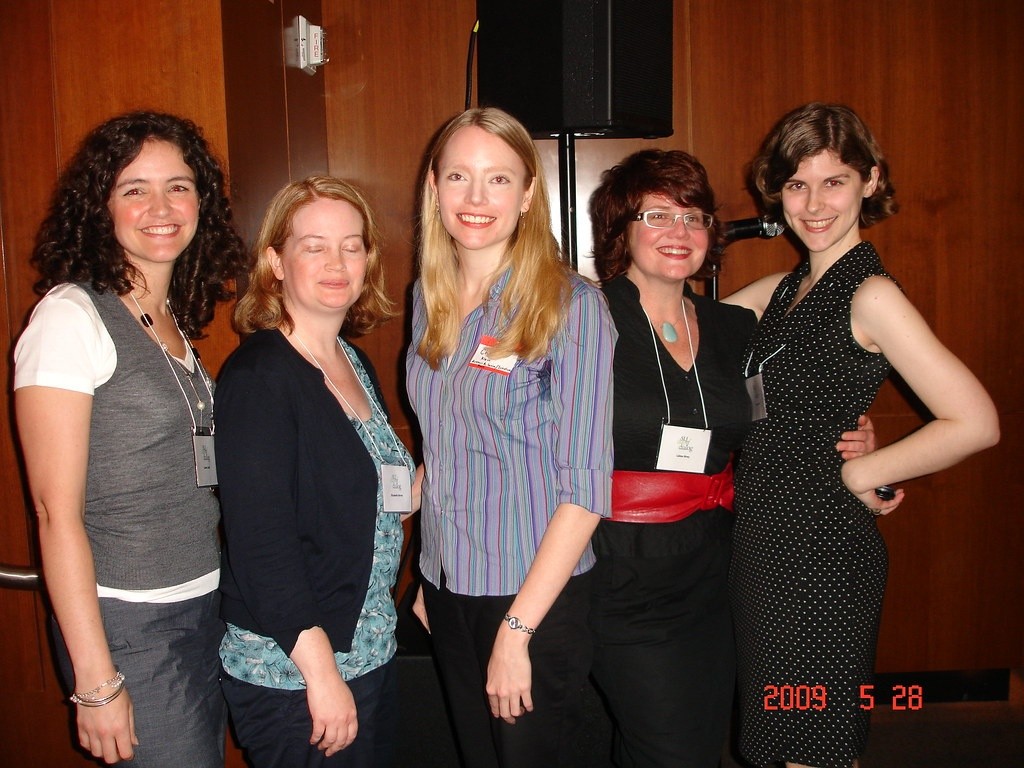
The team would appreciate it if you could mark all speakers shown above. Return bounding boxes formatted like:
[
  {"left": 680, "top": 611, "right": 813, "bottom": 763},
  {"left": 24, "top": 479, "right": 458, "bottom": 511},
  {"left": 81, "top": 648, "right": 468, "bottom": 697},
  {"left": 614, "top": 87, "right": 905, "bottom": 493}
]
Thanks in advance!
[{"left": 476, "top": 0, "right": 673, "bottom": 141}]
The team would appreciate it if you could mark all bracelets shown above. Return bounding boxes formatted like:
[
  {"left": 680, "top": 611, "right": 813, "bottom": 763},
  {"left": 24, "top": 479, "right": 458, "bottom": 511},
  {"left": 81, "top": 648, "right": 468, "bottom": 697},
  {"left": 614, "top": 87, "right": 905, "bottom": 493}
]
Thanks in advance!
[{"left": 69, "top": 665, "right": 125, "bottom": 708}]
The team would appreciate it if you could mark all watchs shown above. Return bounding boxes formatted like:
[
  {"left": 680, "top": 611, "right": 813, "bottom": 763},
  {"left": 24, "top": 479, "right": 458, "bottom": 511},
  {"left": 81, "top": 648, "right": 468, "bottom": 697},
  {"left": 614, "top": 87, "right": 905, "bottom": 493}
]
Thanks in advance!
[{"left": 504, "top": 612, "right": 536, "bottom": 634}]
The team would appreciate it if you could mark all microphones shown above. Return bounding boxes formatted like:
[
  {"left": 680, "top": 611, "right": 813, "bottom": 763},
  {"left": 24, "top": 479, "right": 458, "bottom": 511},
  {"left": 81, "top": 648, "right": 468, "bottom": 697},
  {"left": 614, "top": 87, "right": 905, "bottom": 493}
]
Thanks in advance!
[{"left": 722, "top": 216, "right": 787, "bottom": 241}]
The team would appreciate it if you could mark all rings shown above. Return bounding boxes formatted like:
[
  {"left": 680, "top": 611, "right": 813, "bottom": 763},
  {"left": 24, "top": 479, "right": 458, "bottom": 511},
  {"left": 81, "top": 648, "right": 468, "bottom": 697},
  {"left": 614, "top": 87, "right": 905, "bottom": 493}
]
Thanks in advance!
[{"left": 874, "top": 510, "right": 881, "bottom": 515}]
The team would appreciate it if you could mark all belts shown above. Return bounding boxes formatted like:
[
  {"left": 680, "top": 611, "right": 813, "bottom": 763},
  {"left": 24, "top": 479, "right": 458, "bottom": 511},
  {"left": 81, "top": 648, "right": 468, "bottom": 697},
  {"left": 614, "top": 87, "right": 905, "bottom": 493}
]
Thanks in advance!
[{"left": 603, "top": 452, "right": 735, "bottom": 524}]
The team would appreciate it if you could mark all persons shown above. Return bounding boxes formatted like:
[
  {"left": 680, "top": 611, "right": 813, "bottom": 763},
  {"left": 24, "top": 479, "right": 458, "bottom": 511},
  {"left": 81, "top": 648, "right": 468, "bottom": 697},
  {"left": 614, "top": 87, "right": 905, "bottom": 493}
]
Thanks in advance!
[
  {"left": 717, "top": 101, "right": 1000, "bottom": 768},
  {"left": 399, "top": 108, "right": 620, "bottom": 768},
  {"left": 213, "top": 172, "right": 416, "bottom": 768},
  {"left": 14, "top": 109, "right": 256, "bottom": 768},
  {"left": 586, "top": 147, "right": 875, "bottom": 768}
]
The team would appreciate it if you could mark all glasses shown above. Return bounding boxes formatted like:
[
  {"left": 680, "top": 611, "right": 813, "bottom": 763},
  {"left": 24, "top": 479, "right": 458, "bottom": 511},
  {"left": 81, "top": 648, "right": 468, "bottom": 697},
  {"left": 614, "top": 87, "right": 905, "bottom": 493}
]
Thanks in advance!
[{"left": 630, "top": 210, "right": 714, "bottom": 231}]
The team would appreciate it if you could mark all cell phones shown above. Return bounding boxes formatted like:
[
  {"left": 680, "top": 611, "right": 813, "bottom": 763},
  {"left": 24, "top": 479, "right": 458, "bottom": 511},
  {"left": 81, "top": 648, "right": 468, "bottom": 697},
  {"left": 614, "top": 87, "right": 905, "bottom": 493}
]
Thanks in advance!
[{"left": 876, "top": 486, "right": 896, "bottom": 501}]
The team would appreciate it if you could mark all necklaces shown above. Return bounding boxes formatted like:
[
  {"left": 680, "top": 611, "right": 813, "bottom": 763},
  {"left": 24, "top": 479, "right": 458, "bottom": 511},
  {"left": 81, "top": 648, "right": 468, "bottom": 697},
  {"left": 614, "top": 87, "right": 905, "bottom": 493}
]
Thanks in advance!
[
  {"left": 651, "top": 314, "right": 684, "bottom": 343},
  {"left": 141, "top": 297, "right": 212, "bottom": 433}
]
